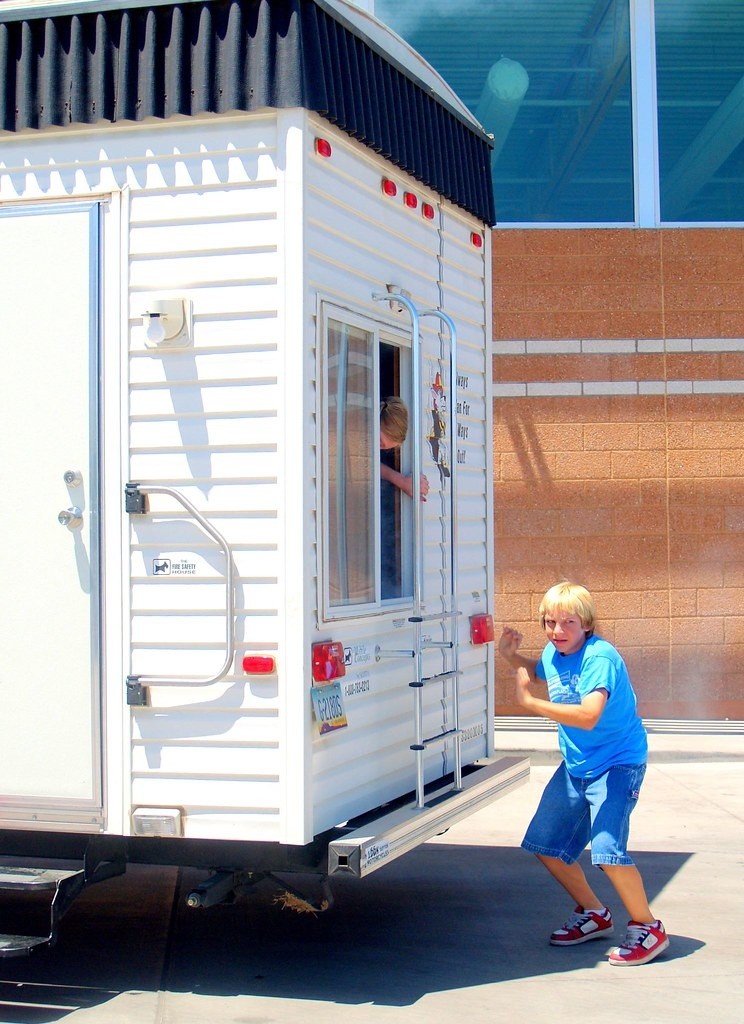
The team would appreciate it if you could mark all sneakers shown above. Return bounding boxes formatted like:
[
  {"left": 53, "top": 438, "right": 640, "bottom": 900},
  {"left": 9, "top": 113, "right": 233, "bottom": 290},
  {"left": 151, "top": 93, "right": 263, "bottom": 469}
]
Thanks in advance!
[
  {"left": 607, "top": 918, "right": 670, "bottom": 965},
  {"left": 549, "top": 904, "right": 615, "bottom": 945}
]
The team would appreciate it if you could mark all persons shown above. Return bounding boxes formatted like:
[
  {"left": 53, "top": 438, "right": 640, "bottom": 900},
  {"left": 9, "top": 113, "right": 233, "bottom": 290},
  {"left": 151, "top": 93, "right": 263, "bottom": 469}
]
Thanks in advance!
[
  {"left": 380, "top": 398, "right": 430, "bottom": 504},
  {"left": 497, "top": 582, "right": 670, "bottom": 966}
]
